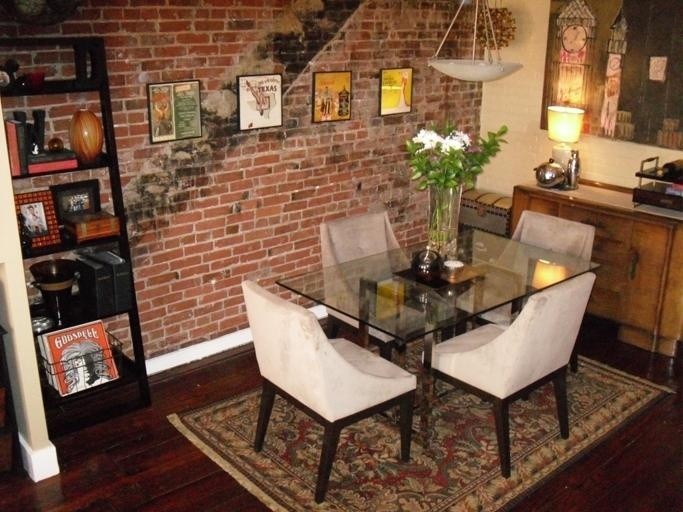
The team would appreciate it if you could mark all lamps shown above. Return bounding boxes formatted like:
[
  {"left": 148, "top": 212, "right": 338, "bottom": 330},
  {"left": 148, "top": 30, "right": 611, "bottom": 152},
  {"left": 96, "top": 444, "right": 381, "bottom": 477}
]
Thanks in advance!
[
  {"left": 547, "top": 104, "right": 585, "bottom": 172},
  {"left": 426, "top": 0, "right": 524, "bottom": 84}
]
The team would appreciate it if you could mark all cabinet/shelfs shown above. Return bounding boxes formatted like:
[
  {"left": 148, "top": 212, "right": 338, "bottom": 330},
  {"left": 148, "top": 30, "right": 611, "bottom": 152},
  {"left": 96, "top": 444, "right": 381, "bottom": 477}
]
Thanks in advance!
[
  {"left": 511, "top": 177, "right": 683, "bottom": 359},
  {"left": 1, "top": 37, "right": 153, "bottom": 441}
]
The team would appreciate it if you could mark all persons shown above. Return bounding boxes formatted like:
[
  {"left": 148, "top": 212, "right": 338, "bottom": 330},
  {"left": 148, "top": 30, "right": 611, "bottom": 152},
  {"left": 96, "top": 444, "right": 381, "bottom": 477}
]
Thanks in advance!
[
  {"left": 60, "top": 341, "right": 113, "bottom": 393},
  {"left": 23, "top": 204, "right": 45, "bottom": 236}
]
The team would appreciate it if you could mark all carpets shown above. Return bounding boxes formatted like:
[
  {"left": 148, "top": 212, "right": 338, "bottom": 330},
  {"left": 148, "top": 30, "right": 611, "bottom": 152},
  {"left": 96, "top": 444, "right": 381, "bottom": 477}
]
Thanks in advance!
[{"left": 166, "top": 355, "right": 666, "bottom": 508}]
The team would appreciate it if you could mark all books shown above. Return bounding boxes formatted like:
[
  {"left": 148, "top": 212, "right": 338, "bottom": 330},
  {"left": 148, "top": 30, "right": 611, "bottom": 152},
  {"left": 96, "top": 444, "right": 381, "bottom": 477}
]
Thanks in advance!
[
  {"left": 38, "top": 317, "right": 120, "bottom": 398},
  {"left": 6, "top": 112, "right": 82, "bottom": 182}
]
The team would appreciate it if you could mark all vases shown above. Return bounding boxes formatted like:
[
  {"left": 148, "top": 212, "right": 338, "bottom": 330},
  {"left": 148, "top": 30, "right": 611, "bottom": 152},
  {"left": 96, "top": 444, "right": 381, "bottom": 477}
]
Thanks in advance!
[{"left": 426, "top": 183, "right": 464, "bottom": 264}]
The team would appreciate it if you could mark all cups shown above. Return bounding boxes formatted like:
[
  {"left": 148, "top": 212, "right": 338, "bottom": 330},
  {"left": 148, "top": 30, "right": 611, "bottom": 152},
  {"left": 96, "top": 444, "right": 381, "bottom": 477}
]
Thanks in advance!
[{"left": 443, "top": 260, "right": 464, "bottom": 279}]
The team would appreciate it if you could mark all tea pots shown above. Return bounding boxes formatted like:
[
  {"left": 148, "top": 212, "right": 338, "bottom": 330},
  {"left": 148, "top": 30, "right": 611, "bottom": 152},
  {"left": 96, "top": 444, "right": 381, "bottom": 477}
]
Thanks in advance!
[
  {"left": 532, "top": 159, "right": 564, "bottom": 189},
  {"left": 410, "top": 249, "right": 442, "bottom": 282}
]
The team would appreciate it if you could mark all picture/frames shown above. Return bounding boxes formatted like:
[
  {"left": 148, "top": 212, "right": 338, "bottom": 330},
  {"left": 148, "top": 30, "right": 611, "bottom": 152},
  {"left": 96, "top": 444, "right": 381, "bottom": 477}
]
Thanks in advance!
[
  {"left": 13, "top": 191, "right": 62, "bottom": 250},
  {"left": 311, "top": 66, "right": 415, "bottom": 125},
  {"left": 49, "top": 179, "right": 102, "bottom": 234},
  {"left": 146, "top": 72, "right": 283, "bottom": 146}
]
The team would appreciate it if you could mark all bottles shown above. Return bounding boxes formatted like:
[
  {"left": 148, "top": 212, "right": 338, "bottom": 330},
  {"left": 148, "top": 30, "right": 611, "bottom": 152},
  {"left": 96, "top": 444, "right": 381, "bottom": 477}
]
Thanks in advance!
[
  {"left": 565, "top": 149, "right": 580, "bottom": 190},
  {"left": 656, "top": 160, "right": 682, "bottom": 179}
]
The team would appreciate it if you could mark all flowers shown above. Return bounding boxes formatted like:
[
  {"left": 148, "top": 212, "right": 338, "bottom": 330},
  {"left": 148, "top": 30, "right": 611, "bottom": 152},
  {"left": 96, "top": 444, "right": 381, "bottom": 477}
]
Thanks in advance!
[{"left": 403, "top": 119, "right": 508, "bottom": 249}]
